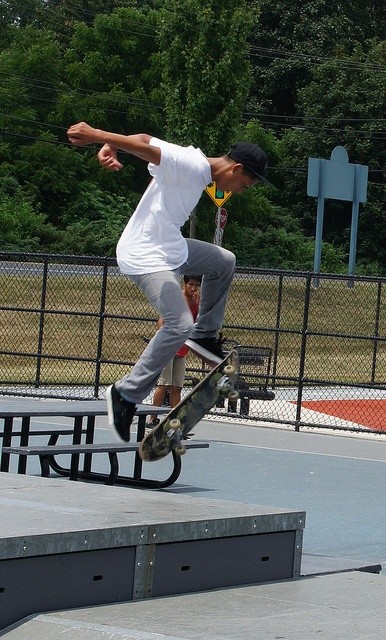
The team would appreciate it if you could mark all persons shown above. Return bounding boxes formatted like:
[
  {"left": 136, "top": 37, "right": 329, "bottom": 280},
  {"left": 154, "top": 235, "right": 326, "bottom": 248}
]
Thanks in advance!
[
  {"left": 68, "top": 121, "right": 269, "bottom": 442},
  {"left": 148, "top": 274, "right": 201, "bottom": 426}
]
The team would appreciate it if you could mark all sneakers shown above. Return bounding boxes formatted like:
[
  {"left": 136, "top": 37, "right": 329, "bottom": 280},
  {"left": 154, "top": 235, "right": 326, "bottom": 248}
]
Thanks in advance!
[
  {"left": 105, "top": 383, "right": 137, "bottom": 441},
  {"left": 184, "top": 337, "right": 226, "bottom": 364},
  {"left": 182, "top": 437, "right": 193, "bottom": 441},
  {"left": 144, "top": 419, "right": 160, "bottom": 430}
]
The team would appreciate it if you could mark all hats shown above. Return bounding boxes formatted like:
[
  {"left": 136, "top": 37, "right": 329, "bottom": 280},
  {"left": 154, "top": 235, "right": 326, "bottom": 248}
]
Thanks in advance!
[{"left": 227, "top": 142, "right": 278, "bottom": 187}]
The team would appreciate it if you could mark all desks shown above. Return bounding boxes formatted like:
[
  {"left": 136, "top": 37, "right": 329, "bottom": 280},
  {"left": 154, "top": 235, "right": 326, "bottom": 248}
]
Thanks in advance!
[{"left": 0, "top": 400, "right": 172, "bottom": 485}]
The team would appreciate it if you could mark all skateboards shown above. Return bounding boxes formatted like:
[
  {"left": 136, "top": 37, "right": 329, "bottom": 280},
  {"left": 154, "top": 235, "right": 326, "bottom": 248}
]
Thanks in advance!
[
  {"left": 136, "top": 424, "right": 195, "bottom": 440},
  {"left": 138, "top": 349, "right": 240, "bottom": 462}
]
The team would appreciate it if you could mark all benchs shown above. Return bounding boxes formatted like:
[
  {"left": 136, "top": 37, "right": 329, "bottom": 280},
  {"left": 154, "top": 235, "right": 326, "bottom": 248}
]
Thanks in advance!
[
  {"left": 0, "top": 426, "right": 86, "bottom": 473},
  {"left": 1, "top": 439, "right": 210, "bottom": 489}
]
